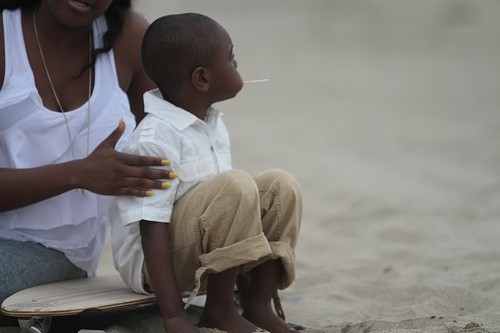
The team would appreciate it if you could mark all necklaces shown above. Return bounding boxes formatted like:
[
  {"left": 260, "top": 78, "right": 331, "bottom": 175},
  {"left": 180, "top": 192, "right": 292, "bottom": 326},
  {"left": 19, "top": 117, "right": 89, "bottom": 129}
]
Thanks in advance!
[{"left": 32, "top": 8, "right": 94, "bottom": 195}]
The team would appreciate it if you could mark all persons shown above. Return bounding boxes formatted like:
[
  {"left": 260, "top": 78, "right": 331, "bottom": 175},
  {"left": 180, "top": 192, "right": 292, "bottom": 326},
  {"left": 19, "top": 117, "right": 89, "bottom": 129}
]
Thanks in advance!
[
  {"left": 107, "top": 12, "right": 304, "bottom": 333},
  {"left": 0, "top": 0, "right": 159, "bottom": 308}
]
